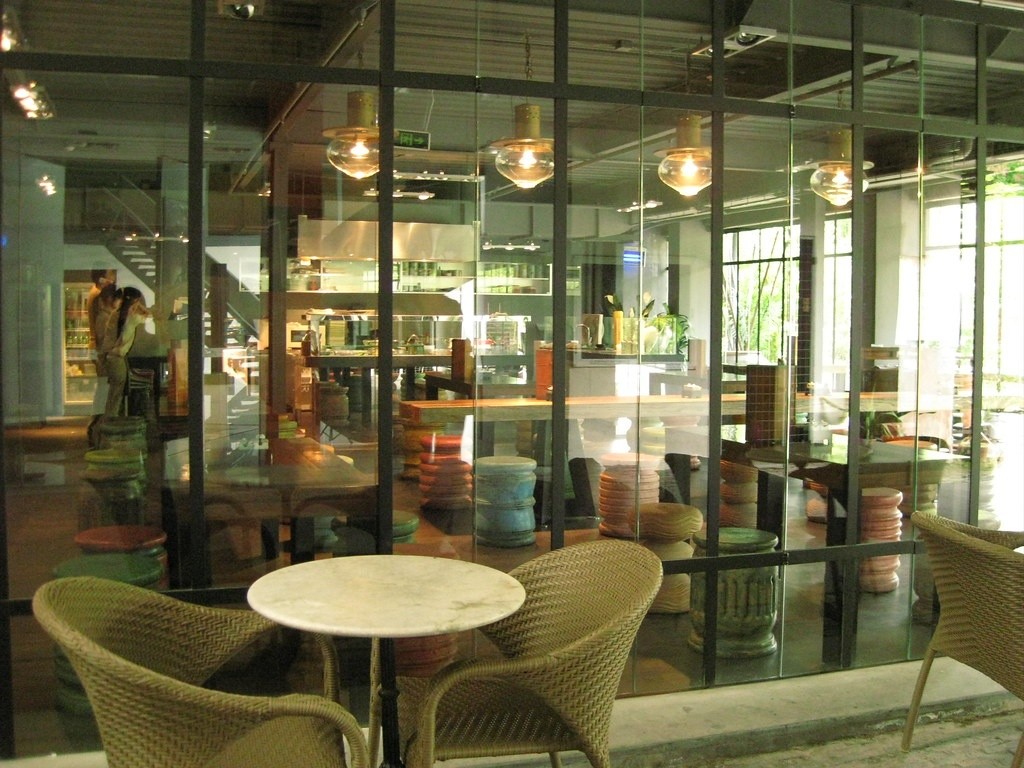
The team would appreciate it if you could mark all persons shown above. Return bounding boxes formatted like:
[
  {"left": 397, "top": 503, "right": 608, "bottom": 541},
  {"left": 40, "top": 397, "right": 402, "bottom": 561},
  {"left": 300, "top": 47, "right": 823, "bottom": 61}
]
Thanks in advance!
[{"left": 86, "top": 261, "right": 153, "bottom": 445}]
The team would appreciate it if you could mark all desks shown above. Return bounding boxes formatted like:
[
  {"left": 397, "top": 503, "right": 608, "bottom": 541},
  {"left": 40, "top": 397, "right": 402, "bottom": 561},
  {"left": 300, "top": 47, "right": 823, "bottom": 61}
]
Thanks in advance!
[
  {"left": 662, "top": 420, "right": 965, "bottom": 669},
  {"left": 248, "top": 555, "right": 527, "bottom": 768},
  {"left": 167, "top": 394, "right": 380, "bottom": 609},
  {"left": 306, "top": 355, "right": 527, "bottom": 427},
  {"left": 401, "top": 390, "right": 894, "bottom": 526}
]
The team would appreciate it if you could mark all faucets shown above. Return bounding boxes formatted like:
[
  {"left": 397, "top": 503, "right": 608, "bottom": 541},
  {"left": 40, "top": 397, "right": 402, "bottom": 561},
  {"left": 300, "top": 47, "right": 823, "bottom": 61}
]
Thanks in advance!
[{"left": 572, "top": 324, "right": 590, "bottom": 341}]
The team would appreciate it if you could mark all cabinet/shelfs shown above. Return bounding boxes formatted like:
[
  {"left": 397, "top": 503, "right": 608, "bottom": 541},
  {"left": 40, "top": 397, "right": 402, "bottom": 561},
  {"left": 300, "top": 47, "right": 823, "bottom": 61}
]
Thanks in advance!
[{"left": 62, "top": 280, "right": 108, "bottom": 405}]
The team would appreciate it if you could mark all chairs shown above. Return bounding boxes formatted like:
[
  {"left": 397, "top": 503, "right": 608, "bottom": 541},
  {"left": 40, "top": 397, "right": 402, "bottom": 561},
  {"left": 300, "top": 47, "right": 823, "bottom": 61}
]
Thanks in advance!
[
  {"left": 29, "top": 576, "right": 369, "bottom": 768},
  {"left": 394, "top": 538, "right": 663, "bottom": 768},
  {"left": 898, "top": 512, "right": 1023, "bottom": 768}
]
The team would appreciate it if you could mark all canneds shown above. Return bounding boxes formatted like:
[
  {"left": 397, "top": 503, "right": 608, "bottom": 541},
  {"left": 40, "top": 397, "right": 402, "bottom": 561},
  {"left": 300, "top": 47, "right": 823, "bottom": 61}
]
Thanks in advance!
[{"left": 67, "top": 336, "right": 90, "bottom": 344}]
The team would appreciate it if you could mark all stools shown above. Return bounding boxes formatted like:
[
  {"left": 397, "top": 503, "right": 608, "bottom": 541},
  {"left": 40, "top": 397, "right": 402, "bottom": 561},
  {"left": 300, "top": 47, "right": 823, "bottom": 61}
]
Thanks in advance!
[{"left": 61, "top": 352, "right": 949, "bottom": 717}]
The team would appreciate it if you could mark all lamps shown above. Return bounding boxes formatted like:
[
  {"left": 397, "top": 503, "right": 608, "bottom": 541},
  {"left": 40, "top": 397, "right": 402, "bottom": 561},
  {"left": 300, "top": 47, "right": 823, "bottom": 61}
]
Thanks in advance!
[{"left": 319, "top": 7, "right": 874, "bottom": 209}]
